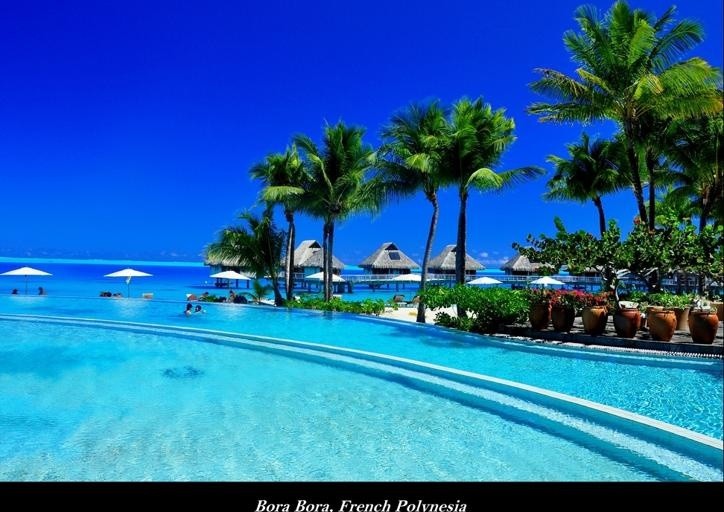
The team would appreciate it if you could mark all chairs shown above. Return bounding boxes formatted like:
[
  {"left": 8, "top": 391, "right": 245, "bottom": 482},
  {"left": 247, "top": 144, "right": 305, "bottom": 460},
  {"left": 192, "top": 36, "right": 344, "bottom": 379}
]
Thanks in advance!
[
  {"left": 397, "top": 302, "right": 407, "bottom": 307},
  {"left": 393, "top": 294, "right": 404, "bottom": 302}
]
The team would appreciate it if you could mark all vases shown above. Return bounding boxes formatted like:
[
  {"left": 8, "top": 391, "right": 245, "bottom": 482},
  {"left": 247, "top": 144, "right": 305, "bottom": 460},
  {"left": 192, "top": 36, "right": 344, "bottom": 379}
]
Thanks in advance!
[{"left": 528, "top": 299, "right": 609, "bottom": 334}]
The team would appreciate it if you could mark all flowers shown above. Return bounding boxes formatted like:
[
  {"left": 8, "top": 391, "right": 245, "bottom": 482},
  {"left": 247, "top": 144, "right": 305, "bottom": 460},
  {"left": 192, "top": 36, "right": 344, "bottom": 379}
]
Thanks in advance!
[{"left": 532, "top": 287, "right": 610, "bottom": 307}]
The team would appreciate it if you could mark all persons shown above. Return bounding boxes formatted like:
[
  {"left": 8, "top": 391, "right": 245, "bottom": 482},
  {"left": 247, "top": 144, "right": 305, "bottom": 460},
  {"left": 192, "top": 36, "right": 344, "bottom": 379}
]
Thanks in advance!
[
  {"left": 38, "top": 287, "right": 45, "bottom": 295},
  {"left": 183, "top": 303, "right": 192, "bottom": 316},
  {"left": 412, "top": 296, "right": 421, "bottom": 308},
  {"left": 229, "top": 290, "right": 236, "bottom": 298},
  {"left": 200, "top": 291, "right": 208, "bottom": 298},
  {"left": 194, "top": 304, "right": 204, "bottom": 314},
  {"left": 12, "top": 289, "right": 17, "bottom": 294},
  {"left": 100, "top": 292, "right": 121, "bottom": 297}
]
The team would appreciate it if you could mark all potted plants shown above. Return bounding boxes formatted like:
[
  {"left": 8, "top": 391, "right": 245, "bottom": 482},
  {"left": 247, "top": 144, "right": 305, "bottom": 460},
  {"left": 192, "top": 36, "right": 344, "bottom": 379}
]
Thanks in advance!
[{"left": 613, "top": 287, "right": 723, "bottom": 344}]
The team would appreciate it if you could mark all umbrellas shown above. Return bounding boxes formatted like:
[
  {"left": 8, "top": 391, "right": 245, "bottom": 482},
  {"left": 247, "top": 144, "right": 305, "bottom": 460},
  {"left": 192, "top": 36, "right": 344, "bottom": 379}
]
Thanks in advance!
[
  {"left": 391, "top": 272, "right": 431, "bottom": 283},
  {"left": 0, "top": 266, "right": 52, "bottom": 295},
  {"left": 467, "top": 276, "right": 503, "bottom": 285},
  {"left": 305, "top": 271, "right": 346, "bottom": 284},
  {"left": 103, "top": 267, "right": 155, "bottom": 298},
  {"left": 209, "top": 270, "right": 251, "bottom": 303},
  {"left": 530, "top": 275, "right": 566, "bottom": 286}
]
